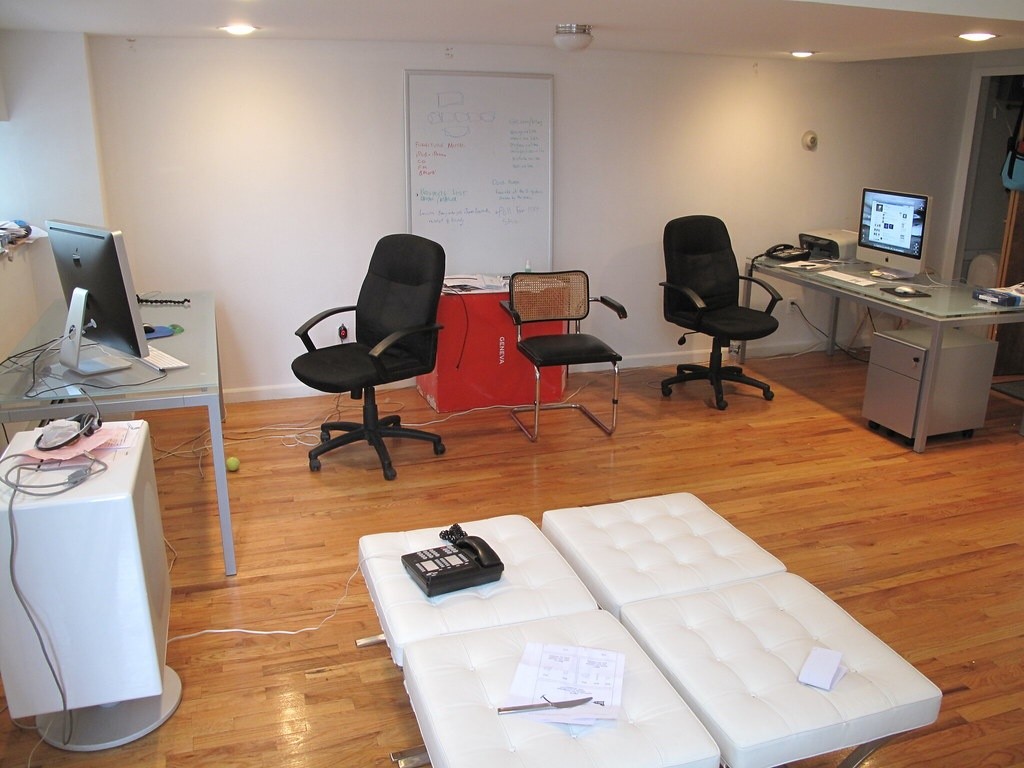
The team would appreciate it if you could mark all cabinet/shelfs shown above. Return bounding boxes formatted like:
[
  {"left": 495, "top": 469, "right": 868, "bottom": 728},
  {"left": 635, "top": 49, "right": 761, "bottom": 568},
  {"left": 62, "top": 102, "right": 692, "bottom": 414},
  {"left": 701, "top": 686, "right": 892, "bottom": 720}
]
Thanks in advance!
[{"left": 859, "top": 326, "right": 1000, "bottom": 436}]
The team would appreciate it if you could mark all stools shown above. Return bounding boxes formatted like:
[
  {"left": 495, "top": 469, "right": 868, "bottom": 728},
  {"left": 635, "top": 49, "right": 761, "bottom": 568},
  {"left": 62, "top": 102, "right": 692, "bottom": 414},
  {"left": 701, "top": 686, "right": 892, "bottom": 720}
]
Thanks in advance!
[{"left": 339, "top": 492, "right": 945, "bottom": 768}]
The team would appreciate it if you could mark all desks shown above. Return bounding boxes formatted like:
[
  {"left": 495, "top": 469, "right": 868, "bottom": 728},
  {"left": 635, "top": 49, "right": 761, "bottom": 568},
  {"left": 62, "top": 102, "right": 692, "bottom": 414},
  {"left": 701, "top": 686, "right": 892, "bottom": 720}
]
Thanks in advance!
[
  {"left": 0, "top": 287, "right": 236, "bottom": 577},
  {"left": 736, "top": 253, "right": 1024, "bottom": 453},
  {"left": 413, "top": 287, "right": 567, "bottom": 416}
]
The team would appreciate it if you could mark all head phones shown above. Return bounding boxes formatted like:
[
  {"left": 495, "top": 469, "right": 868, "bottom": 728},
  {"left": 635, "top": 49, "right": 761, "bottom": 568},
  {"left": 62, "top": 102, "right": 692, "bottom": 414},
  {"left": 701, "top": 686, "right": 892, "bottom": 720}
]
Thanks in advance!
[{"left": 36, "top": 413, "right": 102, "bottom": 451}]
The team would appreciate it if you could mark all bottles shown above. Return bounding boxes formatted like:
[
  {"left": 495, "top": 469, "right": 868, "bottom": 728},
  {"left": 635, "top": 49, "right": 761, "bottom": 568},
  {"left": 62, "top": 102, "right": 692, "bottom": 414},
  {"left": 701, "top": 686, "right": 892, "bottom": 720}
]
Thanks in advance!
[
  {"left": 728, "top": 340, "right": 741, "bottom": 366},
  {"left": 525, "top": 259, "right": 531, "bottom": 272}
]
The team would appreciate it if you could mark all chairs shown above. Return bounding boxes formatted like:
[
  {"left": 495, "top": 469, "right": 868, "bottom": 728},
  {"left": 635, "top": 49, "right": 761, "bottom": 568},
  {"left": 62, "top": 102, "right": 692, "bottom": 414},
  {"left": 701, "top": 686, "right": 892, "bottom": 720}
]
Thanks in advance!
[
  {"left": 499, "top": 270, "right": 627, "bottom": 442},
  {"left": 658, "top": 214, "right": 783, "bottom": 408},
  {"left": 290, "top": 233, "right": 446, "bottom": 480}
]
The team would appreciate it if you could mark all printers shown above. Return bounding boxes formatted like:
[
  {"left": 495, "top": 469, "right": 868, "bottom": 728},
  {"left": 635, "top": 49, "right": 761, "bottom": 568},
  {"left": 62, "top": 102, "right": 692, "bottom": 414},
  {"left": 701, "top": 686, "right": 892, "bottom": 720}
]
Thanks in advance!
[{"left": 798, "top": 229, "right": 859, "bottom": 262}]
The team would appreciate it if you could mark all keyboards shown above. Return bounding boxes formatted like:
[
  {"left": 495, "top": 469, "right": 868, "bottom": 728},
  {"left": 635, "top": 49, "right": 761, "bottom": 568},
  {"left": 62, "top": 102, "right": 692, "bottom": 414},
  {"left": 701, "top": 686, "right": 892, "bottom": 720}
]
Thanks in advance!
[
  {"left": 127, "top": 343, "right": 189, "bottom": 371},
  {"left": 816, "top": 270, "right": 881, "bottom": 286}
]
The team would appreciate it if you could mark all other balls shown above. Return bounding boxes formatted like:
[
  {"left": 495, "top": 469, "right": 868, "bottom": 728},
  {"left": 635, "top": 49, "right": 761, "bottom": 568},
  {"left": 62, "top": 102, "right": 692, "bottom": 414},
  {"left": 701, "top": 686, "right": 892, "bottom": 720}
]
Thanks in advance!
[{"left": 227, "top": 457, "right": 240, "bottom": 471}]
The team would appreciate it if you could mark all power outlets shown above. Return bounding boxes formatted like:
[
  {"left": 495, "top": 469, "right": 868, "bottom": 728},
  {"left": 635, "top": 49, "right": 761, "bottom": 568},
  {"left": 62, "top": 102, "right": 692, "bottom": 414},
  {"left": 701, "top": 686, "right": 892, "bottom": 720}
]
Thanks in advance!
[{"left": 787, "top": 297, "right": 798, "bottom": 313}]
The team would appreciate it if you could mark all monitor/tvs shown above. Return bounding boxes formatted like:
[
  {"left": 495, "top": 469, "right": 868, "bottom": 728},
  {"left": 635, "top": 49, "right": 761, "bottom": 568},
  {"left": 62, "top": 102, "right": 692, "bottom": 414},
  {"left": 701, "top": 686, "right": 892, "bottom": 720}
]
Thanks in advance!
[
  {"left": 855, "top": 188, "right": 935, "bottom": 279},
  {"left": 45, "top": 218, "right": 152, "bottom": 375}
]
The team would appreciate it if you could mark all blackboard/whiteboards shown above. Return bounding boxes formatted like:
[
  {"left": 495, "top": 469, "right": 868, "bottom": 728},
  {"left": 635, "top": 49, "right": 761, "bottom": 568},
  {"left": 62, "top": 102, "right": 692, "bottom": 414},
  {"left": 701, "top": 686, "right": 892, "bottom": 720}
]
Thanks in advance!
[{"left": 404, "top": 68, "right": 554, "bottom": 279}]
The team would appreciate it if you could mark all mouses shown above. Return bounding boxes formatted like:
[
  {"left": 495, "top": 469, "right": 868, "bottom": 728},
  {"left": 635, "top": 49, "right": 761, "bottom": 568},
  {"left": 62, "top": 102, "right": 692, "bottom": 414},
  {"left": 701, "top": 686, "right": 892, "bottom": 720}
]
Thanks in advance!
[
  {"left": 142, "top": 323, "right": 158, "bottom": 333},
  {"left": 895, "top": 285, "right": 923, "bottom": 296}
]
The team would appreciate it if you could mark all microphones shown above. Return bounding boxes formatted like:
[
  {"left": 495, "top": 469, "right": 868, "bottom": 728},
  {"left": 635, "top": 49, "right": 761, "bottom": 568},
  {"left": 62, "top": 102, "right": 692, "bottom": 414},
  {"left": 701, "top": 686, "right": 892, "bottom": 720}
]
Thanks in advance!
[{"left": 79, "top": 387, "right": 113, "bottom": 430}]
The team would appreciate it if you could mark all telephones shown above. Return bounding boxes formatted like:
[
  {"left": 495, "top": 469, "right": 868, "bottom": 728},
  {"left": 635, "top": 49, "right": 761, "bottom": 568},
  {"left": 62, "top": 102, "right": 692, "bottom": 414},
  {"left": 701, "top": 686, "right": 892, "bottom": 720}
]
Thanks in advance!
[
  {"left": 400, "top": 535, "right": 504, "bottom": 597},
  {"left": 765, "top": 244, "right": 810, "bottom": 261}
]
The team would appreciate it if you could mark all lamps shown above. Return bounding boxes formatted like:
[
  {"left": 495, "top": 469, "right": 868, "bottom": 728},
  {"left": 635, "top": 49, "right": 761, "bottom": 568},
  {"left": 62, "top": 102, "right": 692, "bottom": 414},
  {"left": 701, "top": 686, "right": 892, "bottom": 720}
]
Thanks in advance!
[{"left": 553, "top": 24, "right": 593, "bottom": 52}]
